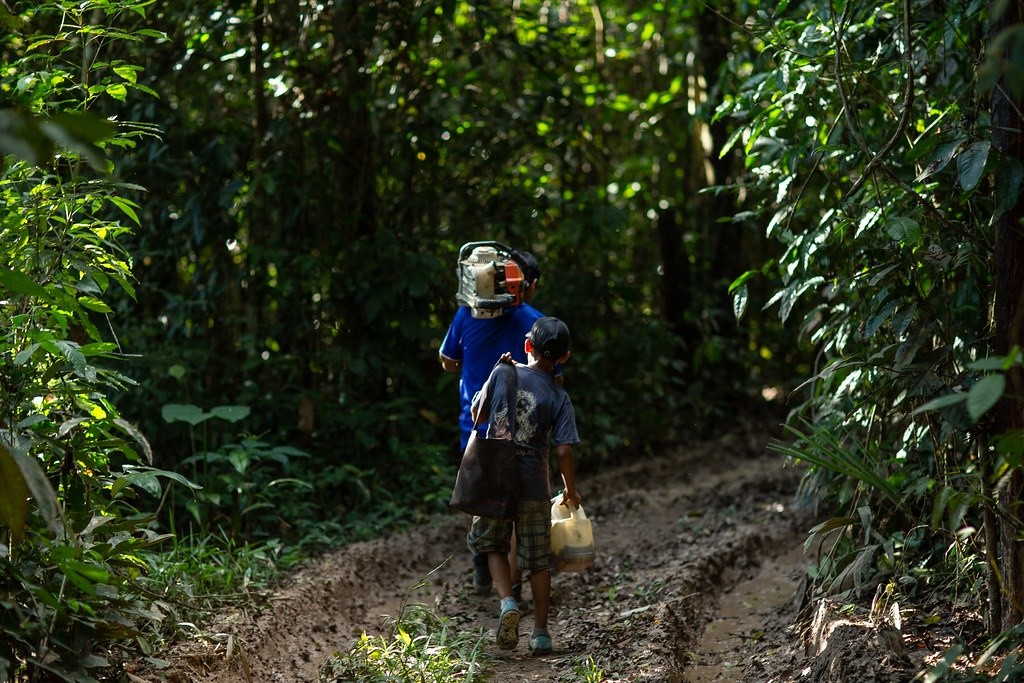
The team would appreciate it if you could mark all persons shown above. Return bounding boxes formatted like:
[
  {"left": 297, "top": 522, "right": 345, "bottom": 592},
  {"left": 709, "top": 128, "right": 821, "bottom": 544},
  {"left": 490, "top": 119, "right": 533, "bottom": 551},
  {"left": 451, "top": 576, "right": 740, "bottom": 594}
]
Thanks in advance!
[
  {"left": 436, "top": 248, "right": 566, "bottom": 613},
  {"left": 470, "top": 315, "right": 583, "bottom": 655}
]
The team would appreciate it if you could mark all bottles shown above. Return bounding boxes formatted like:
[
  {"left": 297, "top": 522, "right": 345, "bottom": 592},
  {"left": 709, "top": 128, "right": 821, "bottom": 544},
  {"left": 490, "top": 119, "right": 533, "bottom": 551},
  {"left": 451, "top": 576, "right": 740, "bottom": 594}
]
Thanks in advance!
[{"left": 550, "top": 490, "right": 597, "bottom": 572}]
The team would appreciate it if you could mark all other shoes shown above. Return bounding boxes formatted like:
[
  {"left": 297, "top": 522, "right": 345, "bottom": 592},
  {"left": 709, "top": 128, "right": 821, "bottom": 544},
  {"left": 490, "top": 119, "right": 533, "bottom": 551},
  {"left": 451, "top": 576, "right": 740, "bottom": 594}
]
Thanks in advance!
[
  {"left": 527, "top": 631, "right": 553, "bottom": 655},
  {"left": 512, "top": 583, "right": 531, "bottom": 617},
  {"left": 496, "top": 599, "right": 521, "bottom": 650},
  {"left": 474, "top": 553, "right": 492, "bottom": 591}
]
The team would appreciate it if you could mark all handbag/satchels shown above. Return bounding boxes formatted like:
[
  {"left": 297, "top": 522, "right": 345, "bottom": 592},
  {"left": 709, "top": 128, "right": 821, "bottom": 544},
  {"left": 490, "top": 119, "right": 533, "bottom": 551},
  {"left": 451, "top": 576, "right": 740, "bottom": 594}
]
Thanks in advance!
[{"left": 449, "top": 360, "right": 523, "bottom": 521}]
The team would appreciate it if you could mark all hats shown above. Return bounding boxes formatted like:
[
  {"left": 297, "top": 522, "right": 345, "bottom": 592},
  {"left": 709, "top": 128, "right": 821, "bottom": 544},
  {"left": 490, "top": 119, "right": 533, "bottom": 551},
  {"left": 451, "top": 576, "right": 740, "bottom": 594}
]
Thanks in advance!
[{"left": 525, "top": 317, "right": 570, "bottom": 357}]
